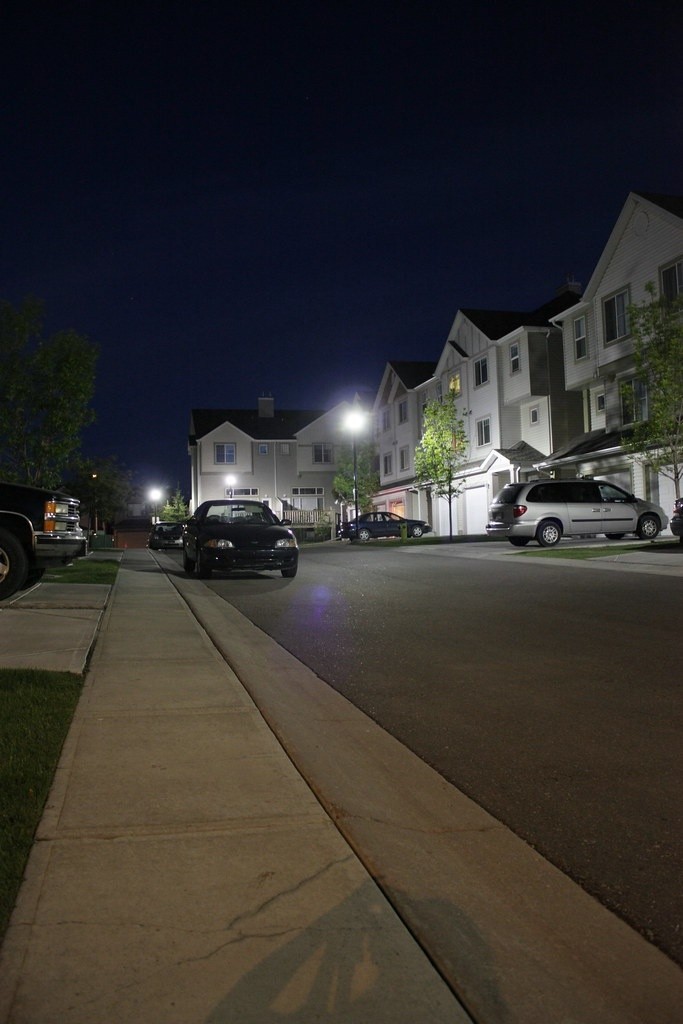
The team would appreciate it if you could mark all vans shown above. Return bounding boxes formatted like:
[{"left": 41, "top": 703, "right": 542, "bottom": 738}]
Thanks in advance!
[{"left": 486, "top": 477, "right": 669, "bottom": 547}]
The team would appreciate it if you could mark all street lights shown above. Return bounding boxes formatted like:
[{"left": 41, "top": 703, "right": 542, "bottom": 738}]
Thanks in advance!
[
  {"left": 228, "top": 477, "right": 235, "bottom": 517},
  {"left": 349, "top": 416, "right": 360, "bottom": 539},
  {"left": 152, "top": 490, "right": 160, "bottom": 523},
  {"left": 92, "top": 474, "right": 97, "bottom": 532}
]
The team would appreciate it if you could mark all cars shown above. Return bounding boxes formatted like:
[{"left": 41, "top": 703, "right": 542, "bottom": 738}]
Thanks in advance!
[
  {"left": 0, "top": 481, "right": 89, "bottom": 608},
  {"left": 670, "top": 498, "right": 683, "bottom": 547},
  {"left": 181, "top": 500, "right": 299, "bottom": 579},
  {"left": 148, "top": 521, "right": 185, "bottom": 550},
  {"left": 337, "top": 512, "right": 432, "bottom": 541}
]
